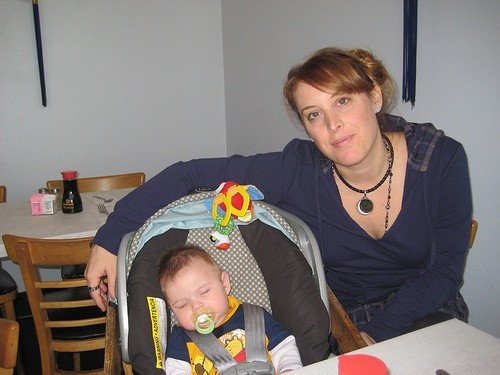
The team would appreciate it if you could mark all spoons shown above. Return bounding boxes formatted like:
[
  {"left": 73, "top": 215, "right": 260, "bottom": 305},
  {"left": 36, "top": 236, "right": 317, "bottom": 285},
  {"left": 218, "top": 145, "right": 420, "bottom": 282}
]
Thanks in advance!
[{"left": 93, "top": 196, "right": 114, "bottom": 202}]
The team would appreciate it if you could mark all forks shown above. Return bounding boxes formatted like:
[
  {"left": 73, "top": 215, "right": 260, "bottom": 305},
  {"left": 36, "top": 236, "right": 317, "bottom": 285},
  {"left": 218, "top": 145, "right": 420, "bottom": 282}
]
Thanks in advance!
[{"left": 97, "top": 203, "right": 110, "bottom": 215}]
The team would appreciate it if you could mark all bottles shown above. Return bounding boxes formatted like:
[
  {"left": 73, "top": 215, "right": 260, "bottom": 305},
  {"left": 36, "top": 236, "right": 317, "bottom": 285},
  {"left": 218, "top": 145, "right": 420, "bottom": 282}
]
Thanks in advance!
[
  {"left": 39, "top": 188, "right": 62, "bottom": 210},
  {"left": 60, "top": 171, "right": 83, "bottom": 214}
]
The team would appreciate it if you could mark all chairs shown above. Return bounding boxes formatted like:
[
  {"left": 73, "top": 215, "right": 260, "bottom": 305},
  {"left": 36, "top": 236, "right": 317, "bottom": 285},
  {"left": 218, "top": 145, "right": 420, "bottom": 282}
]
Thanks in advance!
[{"left": 0, "top": 172, "right": 145, "bottom": 375}]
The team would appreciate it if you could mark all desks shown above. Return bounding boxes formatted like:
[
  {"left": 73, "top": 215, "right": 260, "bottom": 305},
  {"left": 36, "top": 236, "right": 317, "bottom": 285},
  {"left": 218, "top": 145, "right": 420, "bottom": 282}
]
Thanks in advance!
[
  {"left": 280, "top": 318, "right": 500, "bottom": 375},
  {"left": 0, "top": 188, "right": 137, "bottom": 371}
]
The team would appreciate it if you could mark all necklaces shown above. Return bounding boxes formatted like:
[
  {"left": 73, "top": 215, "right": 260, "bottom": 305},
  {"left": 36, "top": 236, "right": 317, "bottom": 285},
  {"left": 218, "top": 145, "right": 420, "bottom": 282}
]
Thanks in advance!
[
  {"left": 333, "top": 132, "right": 394, "bottom": 216},
  {"left": 330, "top": 136, "right": 393, "bottom": 233}
]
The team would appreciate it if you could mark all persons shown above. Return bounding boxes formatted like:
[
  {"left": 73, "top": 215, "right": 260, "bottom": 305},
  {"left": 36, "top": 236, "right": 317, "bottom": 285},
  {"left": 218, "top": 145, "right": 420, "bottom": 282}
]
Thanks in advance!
[
  {"left": 85, "top": 47, "right": 473, "bottom": 360},
  {"left": 159, "top": 243, "right": 303, "bottom": 375}
]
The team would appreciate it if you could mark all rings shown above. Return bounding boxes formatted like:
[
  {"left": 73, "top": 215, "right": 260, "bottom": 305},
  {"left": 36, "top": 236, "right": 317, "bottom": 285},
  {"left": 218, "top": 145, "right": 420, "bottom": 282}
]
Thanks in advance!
[{"left": 89, "top": 285, "right": 99, "bottom": 292}]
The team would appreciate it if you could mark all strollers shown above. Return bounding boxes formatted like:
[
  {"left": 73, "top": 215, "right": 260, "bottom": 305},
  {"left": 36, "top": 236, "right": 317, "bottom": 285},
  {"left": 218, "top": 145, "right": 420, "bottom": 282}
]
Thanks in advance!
[{"left": 107, "top": 181, "right": 331, "bottom": 375}]
